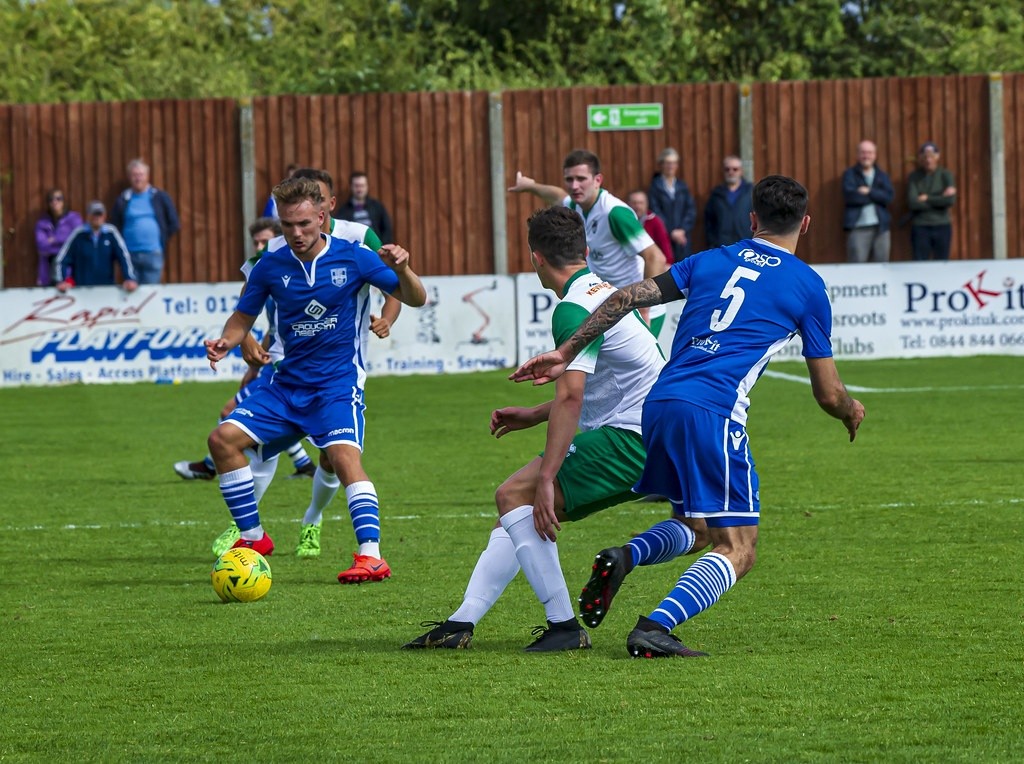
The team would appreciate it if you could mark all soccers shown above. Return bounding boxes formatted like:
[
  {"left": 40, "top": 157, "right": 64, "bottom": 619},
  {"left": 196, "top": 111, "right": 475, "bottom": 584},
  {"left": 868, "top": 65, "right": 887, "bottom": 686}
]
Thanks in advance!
[{"left": 211, "top": 547, "right": 272, "bottom": 603}]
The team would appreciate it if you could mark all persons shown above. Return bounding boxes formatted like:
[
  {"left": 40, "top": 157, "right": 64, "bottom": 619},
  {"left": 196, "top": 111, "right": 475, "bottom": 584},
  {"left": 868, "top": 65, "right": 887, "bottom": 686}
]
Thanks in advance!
[
  {"left": 173, "top": 162, "right": 427, "bottom": 585},
  {"left": 906, "top": 142, "right": 958, "bottom": 259},
  {"left": 841, "top": 138, "right": 897, "bottom": 262},
  {"left": 34, "top": 159, "right": 180, "bottom": 292},
  {"left": 401, "top": 146, "right": 867, "bottom": 660}
]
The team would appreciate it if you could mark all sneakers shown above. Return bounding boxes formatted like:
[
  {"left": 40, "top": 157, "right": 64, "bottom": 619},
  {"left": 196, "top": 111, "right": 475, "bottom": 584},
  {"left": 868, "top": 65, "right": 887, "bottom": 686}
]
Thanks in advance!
[
  {"left": 627, "top": 614, "right": 710, "bottom": 658},
  {"left": 338, "top": 551, "right": 391, "bottom": 585},
  {"left": 174, "top": 460, "right": 217, "bottom": 480},
  {"left": 284, "top": 461, "right": 317, "bottom": 479},
  {"left": 400, "top": 620, "right": 474, "bottom": 650},
  {"left": 230, "top": 530, "right": 275, "bottom": 557},
  {"left": 578, "top": 545, "right": 633, "bottom": 628},
  {"left": 212, "top": 521, "right": 240, "bottom": 557},
  {"left": 524, "top": 617, "right": 592, "bottom": 651},
  {"left": 295, "top": 519, "right": 322, "bottom": 557}
]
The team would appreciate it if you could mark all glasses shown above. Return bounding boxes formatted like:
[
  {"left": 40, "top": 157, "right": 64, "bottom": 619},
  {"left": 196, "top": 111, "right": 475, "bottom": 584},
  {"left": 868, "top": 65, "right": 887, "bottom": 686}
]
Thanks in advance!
[
  {"left": 47, "top": 196, "right": 63, "bottom": 201},
  {"left": 724, "top": 167, "right": 740, "bottom": 171}
]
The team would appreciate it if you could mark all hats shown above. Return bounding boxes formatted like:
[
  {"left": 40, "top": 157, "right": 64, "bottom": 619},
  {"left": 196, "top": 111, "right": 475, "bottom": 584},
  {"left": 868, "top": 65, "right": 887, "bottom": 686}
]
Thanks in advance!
[
  {"left": 88, "top": 202, "right": 105, "bottom": 214},
  {"left": 920, "top": 143, "right": 938, "bottom": 154}
]
